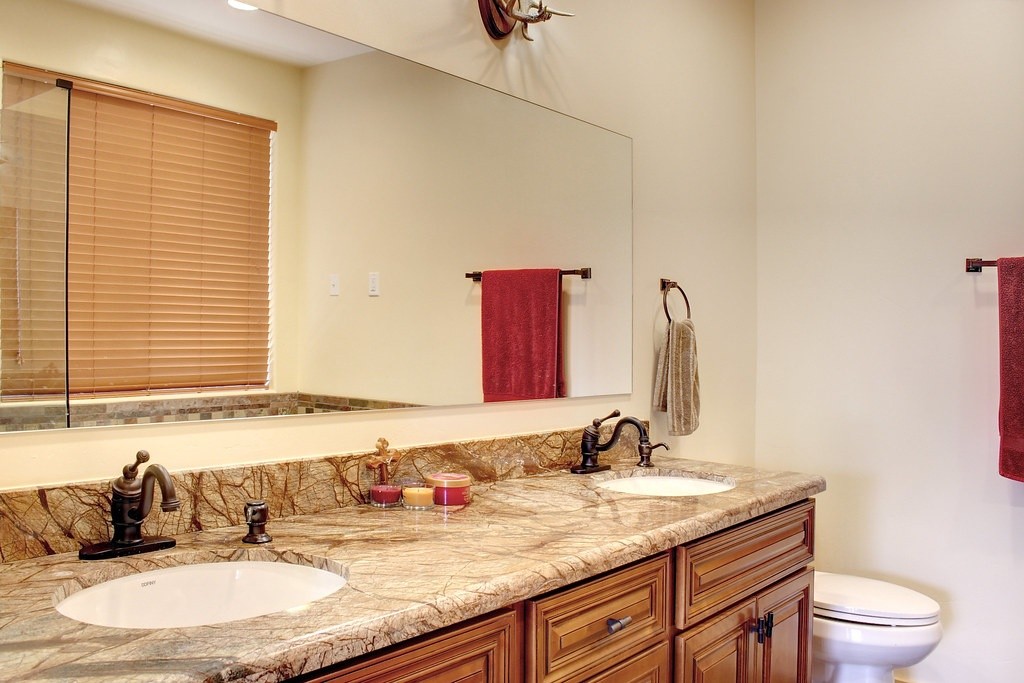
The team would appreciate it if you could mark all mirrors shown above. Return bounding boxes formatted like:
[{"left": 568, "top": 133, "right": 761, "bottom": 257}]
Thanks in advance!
[{"left": 1, "top": 0, "right": 634, "bottom": 433}]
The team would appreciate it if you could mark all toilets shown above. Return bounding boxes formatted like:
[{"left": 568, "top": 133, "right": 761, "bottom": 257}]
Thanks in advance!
[{"left": 812, "top": 569, "right": 945, "bottom": 683}]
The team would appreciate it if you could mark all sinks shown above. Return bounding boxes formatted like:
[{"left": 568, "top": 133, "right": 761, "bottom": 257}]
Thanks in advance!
[
  {"left": 592, "top": 463, "right": 739, "bottom": 499},
  {"left": 50, "top": 537, "right": 358, "bottom": 630}
]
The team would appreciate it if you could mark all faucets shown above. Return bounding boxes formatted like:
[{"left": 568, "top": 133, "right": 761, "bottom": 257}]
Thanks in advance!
[
  {"left": 637, "top": 439, "right": 670, "bottom": 468},
  {"left": 569, "top": 409, "right": 651, "bottom": 476},
  {"left": 109, "top": 448, "right": 181, "bottom": 543}
]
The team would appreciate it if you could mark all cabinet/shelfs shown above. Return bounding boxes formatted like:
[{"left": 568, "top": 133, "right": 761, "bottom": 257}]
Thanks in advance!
[
  {"left": 675, "top": 497, "right": 817, "bottom": 683},
  {"left": 289, "top": 602, "right": 523, "bottom": 682},
  {"left": 520, "top": 549, "right": 675, "bottom": 683}
]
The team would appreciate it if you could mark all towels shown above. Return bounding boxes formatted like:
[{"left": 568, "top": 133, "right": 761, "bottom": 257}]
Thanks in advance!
[
  {"left": 997, "top": 255, "right": 1024, "bottom": 482},
  {"left": 652, "top": 317, "right": 701, "bottom": 440},
  {"left": 480, "top": 269, "right": 568, "bottom": 402}
]
